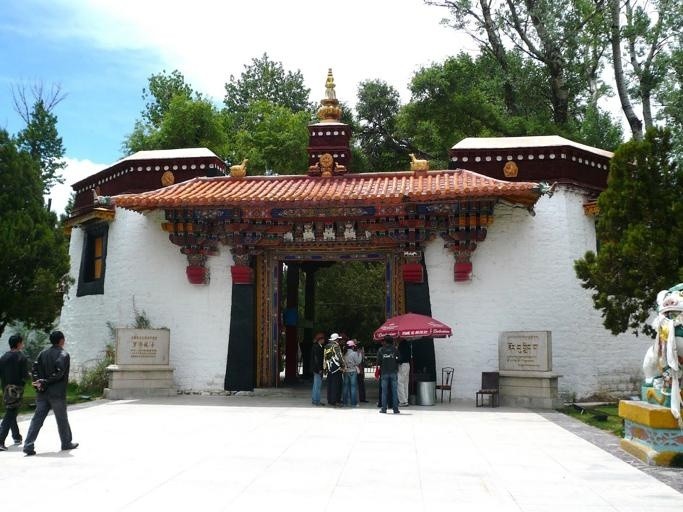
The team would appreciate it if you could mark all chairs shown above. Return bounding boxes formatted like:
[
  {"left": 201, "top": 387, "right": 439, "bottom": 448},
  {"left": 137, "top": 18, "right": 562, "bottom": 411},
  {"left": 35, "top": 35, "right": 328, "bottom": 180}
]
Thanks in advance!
[
  {"left": 474, "top": 371, "right": 499, "bottom": 408},
  {"left": 436, "top": 366, "right": 454, "bottom": 404}
]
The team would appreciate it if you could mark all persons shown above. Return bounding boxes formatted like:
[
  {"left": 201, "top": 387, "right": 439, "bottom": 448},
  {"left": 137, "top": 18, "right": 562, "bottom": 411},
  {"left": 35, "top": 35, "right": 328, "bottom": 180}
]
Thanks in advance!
[
  {"left": 309, "top": 332, "right": 410, "bottom": 414},
  {"left": 0, "top": 332, "right": 28, "bottom": 450},
  {"left": 23, "top": 330, "right": 78, "bottom": 455}
]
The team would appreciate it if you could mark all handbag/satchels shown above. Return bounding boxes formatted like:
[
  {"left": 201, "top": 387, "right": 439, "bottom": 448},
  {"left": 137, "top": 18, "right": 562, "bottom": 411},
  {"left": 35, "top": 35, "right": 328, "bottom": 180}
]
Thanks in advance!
[
  {"left": 2, "top": 384, "right": 24, "bottom": 409},
  {"left": 355, "top": 365, "right": 361, "bottom": 374}
]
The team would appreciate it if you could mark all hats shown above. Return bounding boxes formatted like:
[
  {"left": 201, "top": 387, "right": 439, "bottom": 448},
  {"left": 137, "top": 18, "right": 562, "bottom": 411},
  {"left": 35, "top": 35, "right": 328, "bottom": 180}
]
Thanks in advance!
[
  {"left": 312, "top": 332, "right": 325, "bottom": 343},
  {"left": 328, "top": 332, "right": 342, "bottom": 341},
  {"left": 346, "top": 340, "right": 357, "bottom": 347}
]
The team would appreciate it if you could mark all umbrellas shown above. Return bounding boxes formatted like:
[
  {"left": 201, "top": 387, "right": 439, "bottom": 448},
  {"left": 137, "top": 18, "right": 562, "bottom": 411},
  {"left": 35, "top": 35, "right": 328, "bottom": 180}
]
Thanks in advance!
[{"left": 372, "top": 312, "right": 452, "bottom": 395}]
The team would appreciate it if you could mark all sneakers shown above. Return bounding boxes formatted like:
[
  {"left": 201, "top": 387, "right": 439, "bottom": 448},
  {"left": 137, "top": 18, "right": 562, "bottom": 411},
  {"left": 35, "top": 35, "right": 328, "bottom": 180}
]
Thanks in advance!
[
  {"left": 61, "top": 442, "right": 79, "bottom": 450},
  {"left": 379, "top": 409, "right": 387, "bottom": 413},
  {"left": 360, "top": 399, "right": 370, "bottom": 403},
  {"left": 393, "top": 409, "right": 400, "bottom": 414},
  {"left": 23, "top": 448, "right": 36, "bottom": 455},
  {"left": 328, "top": 401, "right": 360, "bottom": 408},
  {"left": 14, "top": 440, "right": 22, "bottom": 445},
  {"left": 312, "top": 402, "right": 325, "bottom": 407},
  {"left": 0, "top": 443, "right": 8, "bottom": 452},
  {"left": 398, "top": 402, "right": 408, "bottom": 407}
]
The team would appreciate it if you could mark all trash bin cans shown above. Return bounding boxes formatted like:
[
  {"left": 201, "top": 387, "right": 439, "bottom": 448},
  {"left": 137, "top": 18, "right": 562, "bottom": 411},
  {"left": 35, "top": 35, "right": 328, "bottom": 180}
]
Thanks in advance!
[{"left": 415, "top": 366, "right": 437, "bottom": 406}]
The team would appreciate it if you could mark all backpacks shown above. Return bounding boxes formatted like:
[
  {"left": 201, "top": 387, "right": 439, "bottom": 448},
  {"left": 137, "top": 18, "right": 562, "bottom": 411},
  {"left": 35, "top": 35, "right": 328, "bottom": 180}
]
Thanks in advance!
[
  {"left": 382, "top": 353, "right": 396, "bottom": 371},
  {"left": 324, "top": 344, "right": 344, "bottom": 375}
]
